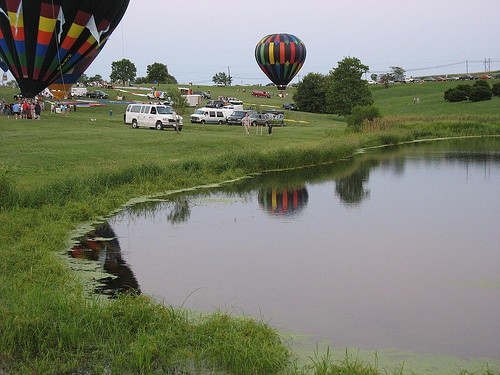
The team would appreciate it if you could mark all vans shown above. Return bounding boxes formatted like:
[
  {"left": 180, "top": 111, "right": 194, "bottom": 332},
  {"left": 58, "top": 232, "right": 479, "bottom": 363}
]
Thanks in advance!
[
  {"left": 124, "top": 103, "right": 184, "bottom": 130},
  {"left": 190, "top": 107, "right": 226, "bottom": 123}
]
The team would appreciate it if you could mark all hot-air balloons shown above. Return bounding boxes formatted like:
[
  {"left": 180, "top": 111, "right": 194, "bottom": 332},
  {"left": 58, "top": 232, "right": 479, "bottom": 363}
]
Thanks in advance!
[
  {"left": 255, "top": 33, "right": 306, "bottom": 99},
  {"left": 0, "top": 0, "right": 131, "bottom": 99}
]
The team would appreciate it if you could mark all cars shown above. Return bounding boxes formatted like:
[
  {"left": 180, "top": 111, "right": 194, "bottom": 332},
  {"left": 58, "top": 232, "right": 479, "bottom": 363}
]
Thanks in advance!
[
  {"left": 423, "top": 77, "right": 435, "bottom": 81},
  {"left": 479, "top": 75, "right": 492, "bottom": 79},
  {"left": 436, "top": 77, "right": 446, "bottom": 81},
  {"left": 251, "top": 90, "right": 271, "bottom": 99},
  {"left": 366, "top": 78, "right": 418, "bottom": 84},
  {"left": 14, "top": 93, "right": 23, "bottom": 100},
  {"left": 493, "top": 74, "right": 500, "bottom": 79},
  {"left": 227, "top": 109, "right": 274, "bottom": 126},
  {"left": 447, "top": 76, "right": 459, "bottom": 80},
  {"left": 192, "top": 91, "right": 211, "bottom": 98},
  {"left": 85, "top": 89, "right": 109, "bottom": 98},
  {"left": 283, "top": 103, "right": 298, "bottom": 111},
  {"left": 459, "top": 74, "right": 478, "bottom": 79}
]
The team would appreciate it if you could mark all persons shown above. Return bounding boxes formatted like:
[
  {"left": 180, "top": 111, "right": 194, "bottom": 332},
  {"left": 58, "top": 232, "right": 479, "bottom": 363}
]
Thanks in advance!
[
  {"left": 0, "top": 101, "right": 40, "bottom": 120},
  {"left": 242, "top": 113, "right": 251, "bottom": 135}
]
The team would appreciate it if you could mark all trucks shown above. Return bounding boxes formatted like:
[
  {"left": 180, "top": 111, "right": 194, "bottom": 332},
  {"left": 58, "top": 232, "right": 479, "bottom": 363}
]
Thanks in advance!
[
  {"left": 71, "top": 88, "right": 89, "bottom": 97},
  {"left": 206, "top": 97, "right": 244, "bottom": 111},
  {"left": 265, "top": 111, "right": 287, "bottom": 127},
  {"left": 147, "top": 91, "right": 168, "bottom": 99}
]
